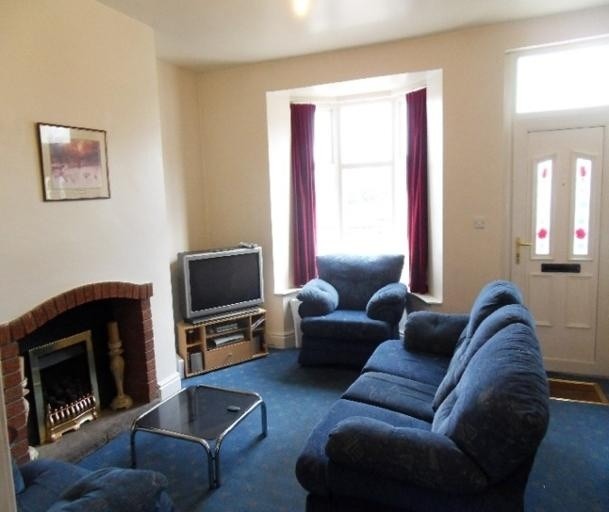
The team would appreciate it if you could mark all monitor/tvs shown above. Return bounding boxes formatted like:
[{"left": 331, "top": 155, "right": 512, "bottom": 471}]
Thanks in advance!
[{"left": 176, "top": 247, "right": 264, "bottom": 326}]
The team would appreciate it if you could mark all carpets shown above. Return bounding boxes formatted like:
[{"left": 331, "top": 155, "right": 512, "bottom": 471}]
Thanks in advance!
[{"left": 547, "top": 377, "right": 608, "bottom": 406}]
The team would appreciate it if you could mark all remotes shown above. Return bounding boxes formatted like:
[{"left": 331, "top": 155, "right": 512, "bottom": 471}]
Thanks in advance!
[{"left": 238, "top": 240, "right": 258, "bottom": 247}]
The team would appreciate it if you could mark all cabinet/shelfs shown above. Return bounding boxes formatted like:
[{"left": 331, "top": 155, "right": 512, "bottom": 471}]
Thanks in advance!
[{"left": 175, "top": 307, "right": 270, "bottom": 379}]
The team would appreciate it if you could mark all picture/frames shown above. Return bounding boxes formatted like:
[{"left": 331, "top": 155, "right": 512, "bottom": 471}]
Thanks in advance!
[{"left": 36, "top": 120, "right": 113, "bottom": 202}]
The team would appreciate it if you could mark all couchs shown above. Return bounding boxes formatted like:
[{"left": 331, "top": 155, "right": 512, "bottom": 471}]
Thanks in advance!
[{"left": 297, "top": 280, "right": 553, "bottom": 512}]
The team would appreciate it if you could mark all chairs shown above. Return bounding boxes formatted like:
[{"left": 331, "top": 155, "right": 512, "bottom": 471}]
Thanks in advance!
[
  {"left": 294, "top": 249, "right": 410, "bottom": 369},
  {"left": 13, "top": 455, "right": 174, "bottom": 512}
]
